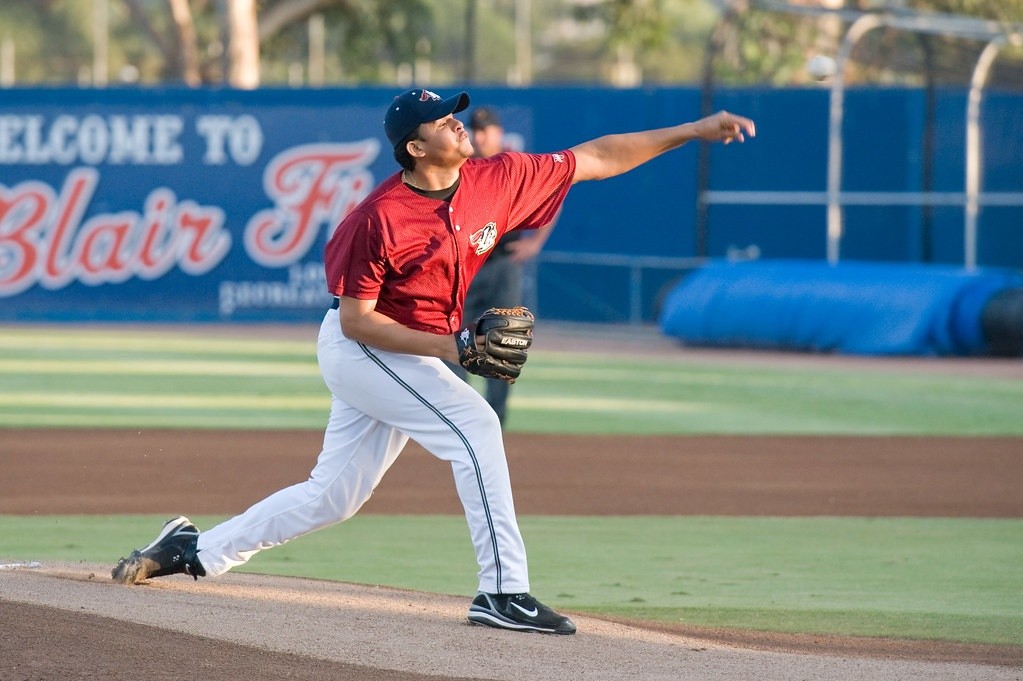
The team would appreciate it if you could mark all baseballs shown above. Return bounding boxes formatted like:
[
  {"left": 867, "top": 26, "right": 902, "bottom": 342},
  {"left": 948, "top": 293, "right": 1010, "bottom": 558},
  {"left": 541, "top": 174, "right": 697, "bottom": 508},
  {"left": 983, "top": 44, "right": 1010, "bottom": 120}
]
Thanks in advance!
[{"left": 805, "top": 54, "right": 836, "bottom": 81}]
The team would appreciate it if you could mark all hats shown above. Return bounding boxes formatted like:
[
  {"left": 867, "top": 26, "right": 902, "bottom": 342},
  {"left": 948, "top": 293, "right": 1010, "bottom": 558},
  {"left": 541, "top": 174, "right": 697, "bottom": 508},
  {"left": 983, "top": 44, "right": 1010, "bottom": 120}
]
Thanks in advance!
[
  {"left": 466, "top": 107, "right": 497, "bottom": 128},
  {"left": 384, "top": 88, "right": 470, "bottom": 149}
]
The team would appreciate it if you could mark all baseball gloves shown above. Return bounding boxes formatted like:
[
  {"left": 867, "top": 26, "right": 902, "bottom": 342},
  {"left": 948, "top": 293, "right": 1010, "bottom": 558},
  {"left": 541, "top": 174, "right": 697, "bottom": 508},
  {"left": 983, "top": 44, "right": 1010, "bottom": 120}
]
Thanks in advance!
[{"left": 453, "top": 306, "right": 535, "bottom": 385}]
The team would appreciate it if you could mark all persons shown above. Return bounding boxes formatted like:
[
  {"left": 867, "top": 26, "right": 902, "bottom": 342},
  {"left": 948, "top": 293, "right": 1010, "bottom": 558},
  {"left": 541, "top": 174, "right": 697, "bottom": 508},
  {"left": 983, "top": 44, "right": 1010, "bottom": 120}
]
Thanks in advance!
[
  {"left": 114, "top": 89, "right": 758, "bottom": 637},
  {"left": 441, "top": 105, "right": 565, "bottom": 430}
]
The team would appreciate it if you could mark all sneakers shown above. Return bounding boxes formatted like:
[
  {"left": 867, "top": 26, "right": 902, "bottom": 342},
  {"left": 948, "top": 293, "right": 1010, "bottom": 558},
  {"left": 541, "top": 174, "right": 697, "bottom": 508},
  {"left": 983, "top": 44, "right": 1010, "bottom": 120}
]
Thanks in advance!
[
  {"left": 467, "top": 591, "right": 576, "bottom": 636},
  {"left": 111, "top": 515, "right": 206, "bottom": 585}
]
